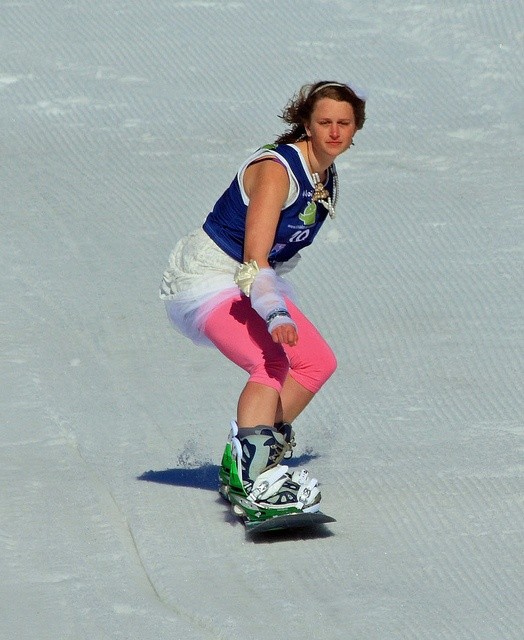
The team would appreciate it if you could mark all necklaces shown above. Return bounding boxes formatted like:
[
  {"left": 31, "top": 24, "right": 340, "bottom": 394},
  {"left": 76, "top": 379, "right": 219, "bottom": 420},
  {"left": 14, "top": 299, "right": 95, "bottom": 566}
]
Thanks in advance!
[{"left": 303, "top": 139, "right": 333, "bottom": 202}]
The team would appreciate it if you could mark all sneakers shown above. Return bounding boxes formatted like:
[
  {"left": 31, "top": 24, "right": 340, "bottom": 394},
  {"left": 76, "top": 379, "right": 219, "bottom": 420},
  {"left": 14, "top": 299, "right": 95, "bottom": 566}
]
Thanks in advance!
[{"left": 236, "top": 422, "right": 322, "bottom": 511}]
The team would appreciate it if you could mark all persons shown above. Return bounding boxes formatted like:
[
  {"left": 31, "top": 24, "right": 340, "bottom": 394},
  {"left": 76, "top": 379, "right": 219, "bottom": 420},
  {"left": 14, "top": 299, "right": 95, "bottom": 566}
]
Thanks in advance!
[{"left": 157, "top": 78, "right": 368, "bottom": 512}]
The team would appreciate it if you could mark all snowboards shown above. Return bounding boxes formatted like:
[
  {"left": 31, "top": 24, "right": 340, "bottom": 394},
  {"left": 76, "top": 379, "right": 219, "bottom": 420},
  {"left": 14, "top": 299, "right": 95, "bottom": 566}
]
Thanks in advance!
[{"left": 218, "top": 483, "right": 336, "bottom": 535}]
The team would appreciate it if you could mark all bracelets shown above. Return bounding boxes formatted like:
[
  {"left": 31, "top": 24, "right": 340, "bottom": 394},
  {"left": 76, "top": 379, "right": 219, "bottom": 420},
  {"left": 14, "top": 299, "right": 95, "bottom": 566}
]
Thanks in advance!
[{"left": 264, "top": 309, "right": 293, "bottom": 328}]
